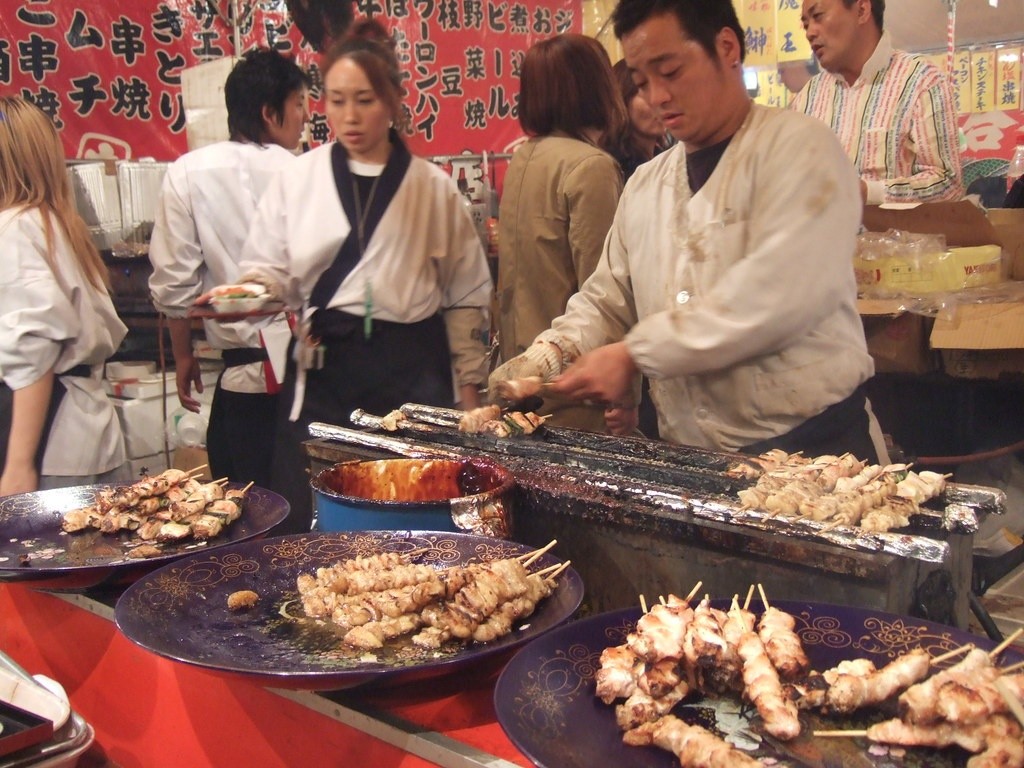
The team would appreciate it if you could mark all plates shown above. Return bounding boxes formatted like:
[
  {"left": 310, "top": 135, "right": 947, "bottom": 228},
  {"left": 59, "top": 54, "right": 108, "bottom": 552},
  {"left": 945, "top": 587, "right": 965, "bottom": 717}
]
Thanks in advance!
[
  {"left": 493, "top": 601, "right": 1024, "bottom": 768},
  {"left": 138, "top": 371, "right": 177, "bottom": 384},
  {"left": 110, "top": 533, "right": 587, "bottom": 677},
  {"left": 0, "top": 478, "right": 291, "bottom": 570}
]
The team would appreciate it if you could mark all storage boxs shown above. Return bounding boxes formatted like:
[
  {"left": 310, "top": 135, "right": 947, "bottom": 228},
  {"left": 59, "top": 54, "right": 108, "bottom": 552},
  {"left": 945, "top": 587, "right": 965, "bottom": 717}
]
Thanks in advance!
[
  {"left": 179, "top": 55, "right": 311, "bottom": 160},
  {"left": 854, "top": 191, "right": 1024, "bottom": 382},
  {"left": 94, "top": 330, "right": 228, "bottom": 484}
]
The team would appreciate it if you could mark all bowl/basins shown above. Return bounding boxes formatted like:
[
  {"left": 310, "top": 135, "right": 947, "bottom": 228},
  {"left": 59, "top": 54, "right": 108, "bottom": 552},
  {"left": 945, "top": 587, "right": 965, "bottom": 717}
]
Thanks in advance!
[
  {"left": 210, "top": 291, "right": 269, "bottom": 314},
  {"left": 177, "top": 413, "right": 208, "bottom": 445}
]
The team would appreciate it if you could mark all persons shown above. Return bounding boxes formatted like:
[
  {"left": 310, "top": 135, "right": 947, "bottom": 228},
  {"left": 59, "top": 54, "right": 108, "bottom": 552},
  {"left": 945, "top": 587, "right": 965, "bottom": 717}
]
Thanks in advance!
[
  {"left": 194, "top": 17, "right": 494, "bottom": 535},
  {"left": 497, "top": 33, "right": 681, "bottom": 441},
  {"left": 785, "top": 0, "right": 964, "bottom": 207},
  {"left": 147, "top": 47, "right": 311, "bottom": 534},
  {"left": 0, "top": 95, "right": 130, "bottom": 499},
  {"left": 488, "top": 0, "right": 893, "bottom": 467}
]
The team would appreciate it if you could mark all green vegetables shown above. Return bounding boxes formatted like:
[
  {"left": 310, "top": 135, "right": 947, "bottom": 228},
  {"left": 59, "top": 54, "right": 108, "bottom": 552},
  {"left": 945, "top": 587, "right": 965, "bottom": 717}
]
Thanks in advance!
[{"left": 226, "top": 293, "right": 258, "bottom": 298}]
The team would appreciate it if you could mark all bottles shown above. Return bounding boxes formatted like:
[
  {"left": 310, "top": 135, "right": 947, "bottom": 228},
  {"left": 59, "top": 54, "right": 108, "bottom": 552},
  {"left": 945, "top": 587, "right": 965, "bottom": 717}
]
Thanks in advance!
[{"left": 1006, "top": 134, "right": 1024, "bottom": 194}]
